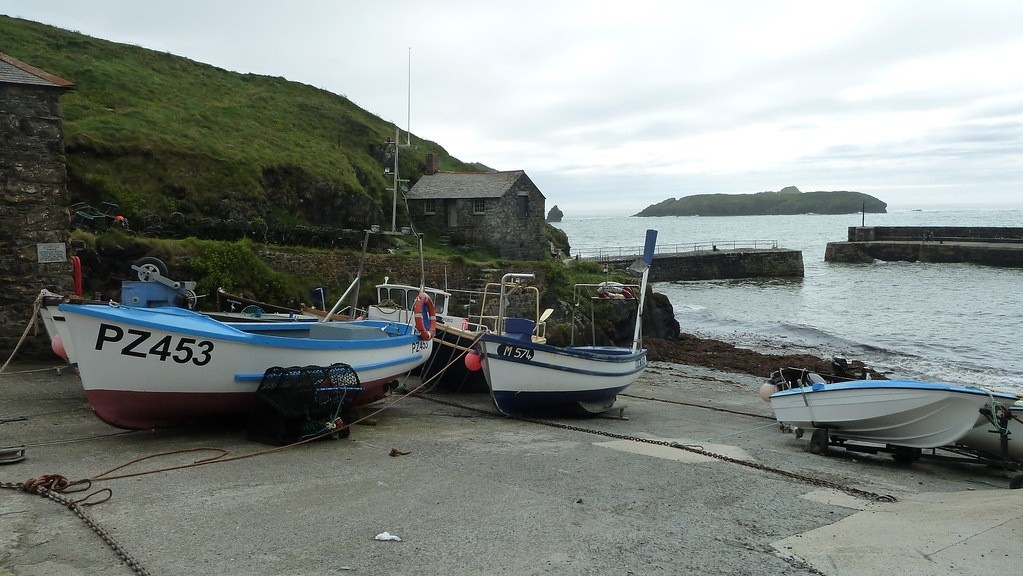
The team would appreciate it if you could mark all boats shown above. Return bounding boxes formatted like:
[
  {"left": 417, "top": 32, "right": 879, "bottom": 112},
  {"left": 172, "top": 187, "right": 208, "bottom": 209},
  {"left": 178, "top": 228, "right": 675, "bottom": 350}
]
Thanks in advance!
[
  {"left": 34, "top": 45, "right": 440, "bottom": 449},
  {"left": 760, "top": 354, "right": 1023, "bottom": 490},
  {"left": 471, "top": 228, "right": 658, "bottom": 421},
  {"left": 371, "top": 269, "right": 554, "bottom": 398}
]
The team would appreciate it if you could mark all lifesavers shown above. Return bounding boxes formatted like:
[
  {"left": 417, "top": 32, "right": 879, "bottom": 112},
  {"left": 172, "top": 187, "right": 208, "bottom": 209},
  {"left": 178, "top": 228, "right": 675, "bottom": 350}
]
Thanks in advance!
[{"left": 414, "top": 291, "right": 436, "bottom": 341}]
